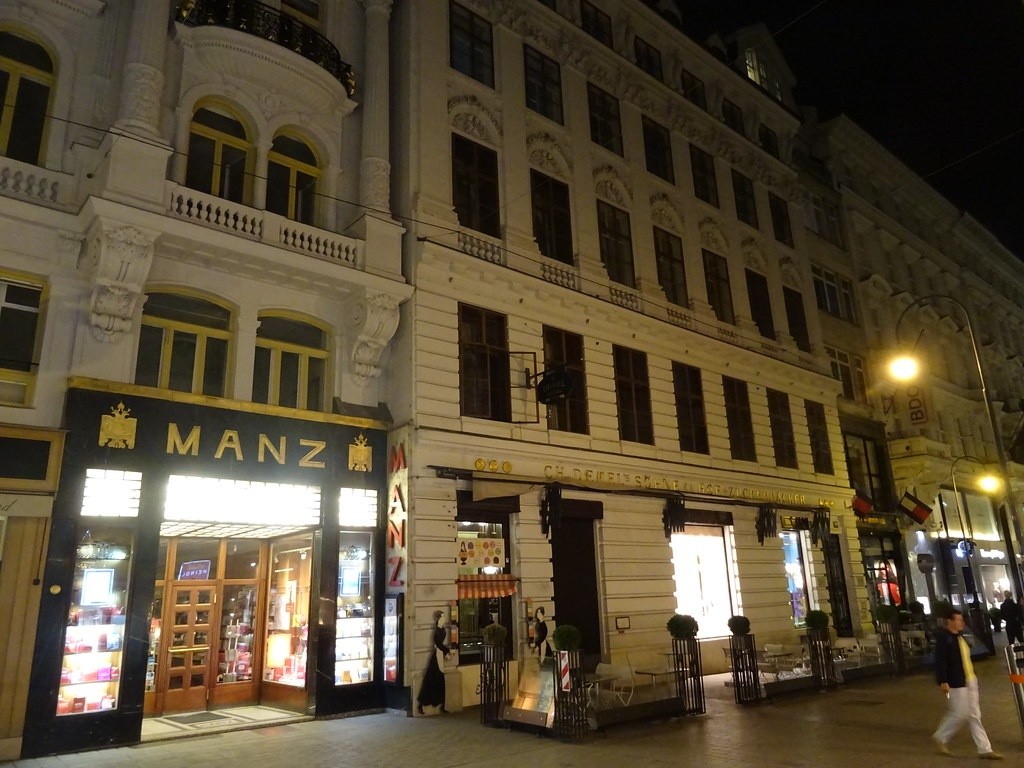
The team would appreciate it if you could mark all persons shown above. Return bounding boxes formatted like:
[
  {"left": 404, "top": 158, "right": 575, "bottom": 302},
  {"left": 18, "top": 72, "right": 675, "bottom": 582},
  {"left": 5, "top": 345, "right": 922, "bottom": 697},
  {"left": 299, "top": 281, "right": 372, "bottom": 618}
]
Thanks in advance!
[{"left": 928, "top": 609, "right": 1005, "bottom": 760}]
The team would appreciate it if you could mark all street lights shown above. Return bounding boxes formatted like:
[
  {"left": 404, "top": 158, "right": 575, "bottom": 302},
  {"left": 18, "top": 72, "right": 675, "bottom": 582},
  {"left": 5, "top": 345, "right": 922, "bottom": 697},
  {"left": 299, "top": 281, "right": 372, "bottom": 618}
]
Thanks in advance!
[
  {"left": 888, "top": 294, "right": 1024, "bottom": 559},
  {"left": 950, "top": 454, "right": 998, "bottom": 656}
]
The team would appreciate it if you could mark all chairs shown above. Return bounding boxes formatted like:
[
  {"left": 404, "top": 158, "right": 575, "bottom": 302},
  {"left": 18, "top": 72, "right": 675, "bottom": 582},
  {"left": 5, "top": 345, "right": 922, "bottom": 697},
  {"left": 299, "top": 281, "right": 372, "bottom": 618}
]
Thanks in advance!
[
  {"left": 833, "top": 647, "right": 847, "bottom": 660},
  {"left": 722, "top": 643, "right": 793, "bottom": 681},
  {"left": 659, "top": 653, "right": 689, "bottom": 685},
  {"left": 800, "top": 635, "right": 810, "bottom": 657}
]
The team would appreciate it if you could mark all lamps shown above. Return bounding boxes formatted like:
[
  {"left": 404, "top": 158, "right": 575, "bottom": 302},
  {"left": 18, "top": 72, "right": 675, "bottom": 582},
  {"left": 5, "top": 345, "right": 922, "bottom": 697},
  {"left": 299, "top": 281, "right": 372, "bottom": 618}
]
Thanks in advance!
[
  {"left": 958, "top": 538, "right": 976, "bottom": 553},
  {"left": 915, "top": 529, "right": 926, "bottom": 533}
]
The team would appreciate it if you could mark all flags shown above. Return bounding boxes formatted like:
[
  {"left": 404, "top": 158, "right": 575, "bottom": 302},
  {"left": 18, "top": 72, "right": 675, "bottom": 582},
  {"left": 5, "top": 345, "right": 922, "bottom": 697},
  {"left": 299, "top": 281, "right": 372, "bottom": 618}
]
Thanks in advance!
[
  {"left": 898, "top": 491, "right": 932, "bottom": 524},
  {"left": 854, "top": 490, "right": 876, "bottom": 518}
]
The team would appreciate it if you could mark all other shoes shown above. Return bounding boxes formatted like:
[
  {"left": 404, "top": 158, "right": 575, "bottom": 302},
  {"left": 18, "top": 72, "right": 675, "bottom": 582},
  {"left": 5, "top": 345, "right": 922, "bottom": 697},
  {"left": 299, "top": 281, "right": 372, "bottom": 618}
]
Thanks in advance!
[
  {"left": 979, "top": 752, "right": 1006, "bottom": 760},
  {"left": 932, "top": 737, "right": 951, "bottom": 755}
]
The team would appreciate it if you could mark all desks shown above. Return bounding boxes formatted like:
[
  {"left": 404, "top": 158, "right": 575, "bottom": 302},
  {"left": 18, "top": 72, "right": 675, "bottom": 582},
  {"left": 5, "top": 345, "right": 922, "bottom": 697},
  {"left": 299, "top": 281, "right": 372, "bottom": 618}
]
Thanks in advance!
[
  {"left": 575, "top": 673, "right": 623, "bottom": 696},
  {"left": 635, "top": 667, "right": 690, "bottom": 687}
]
